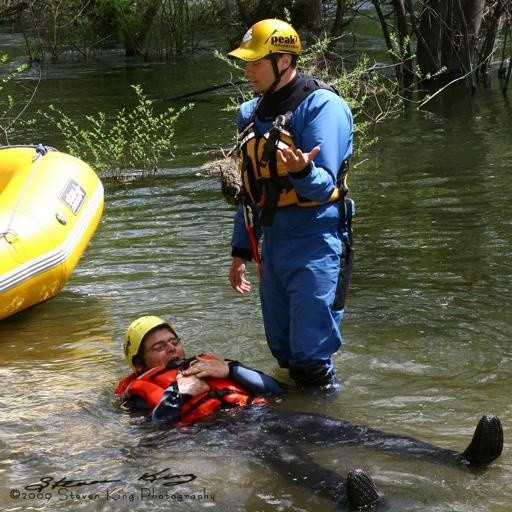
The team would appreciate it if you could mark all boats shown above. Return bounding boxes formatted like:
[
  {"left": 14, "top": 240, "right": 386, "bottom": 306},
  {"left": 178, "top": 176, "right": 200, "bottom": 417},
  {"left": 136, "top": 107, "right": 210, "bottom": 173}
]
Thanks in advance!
[{"left": 1, "top": 141, "right": 108, "bottom": 325}]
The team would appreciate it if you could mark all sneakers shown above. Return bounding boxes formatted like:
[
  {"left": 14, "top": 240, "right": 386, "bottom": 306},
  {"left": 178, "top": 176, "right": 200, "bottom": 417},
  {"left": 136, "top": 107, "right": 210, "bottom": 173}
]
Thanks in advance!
[
  {"left": 447, "top": 415, "right": 502, "bottom": 476},
  {"left": 347, "top": 468, "right": 378, "bottom": 512}
]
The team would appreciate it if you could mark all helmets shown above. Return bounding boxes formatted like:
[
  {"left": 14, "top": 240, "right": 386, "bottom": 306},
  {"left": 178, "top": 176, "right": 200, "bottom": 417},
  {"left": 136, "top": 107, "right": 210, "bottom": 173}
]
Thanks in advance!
[
  {"left": 228, "top": 19, "right": 301, "bottom": 62},
  {"left": 124, "top": 316, "right": 168, "bottom": 376}
]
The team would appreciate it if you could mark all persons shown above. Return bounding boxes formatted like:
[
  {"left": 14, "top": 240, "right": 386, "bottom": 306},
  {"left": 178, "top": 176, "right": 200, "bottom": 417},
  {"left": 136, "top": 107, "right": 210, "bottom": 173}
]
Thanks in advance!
[
  {"left": 114, "top": 315, "right": 503, "bottom": 512},
  {"left": 226, "top": 17, "right": 356, "bottom": 401}
]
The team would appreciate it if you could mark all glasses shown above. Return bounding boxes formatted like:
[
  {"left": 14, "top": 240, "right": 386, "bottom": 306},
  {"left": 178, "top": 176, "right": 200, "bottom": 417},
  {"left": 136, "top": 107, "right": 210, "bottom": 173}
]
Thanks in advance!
[{"left": 146, "top": 337, "right": 181, "bottom": 352}]
